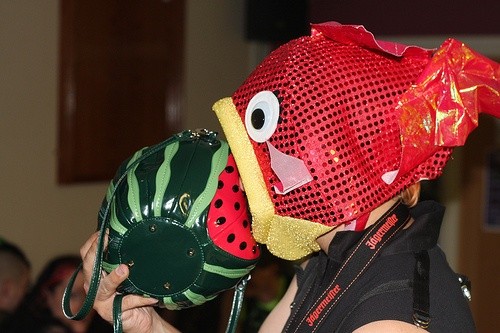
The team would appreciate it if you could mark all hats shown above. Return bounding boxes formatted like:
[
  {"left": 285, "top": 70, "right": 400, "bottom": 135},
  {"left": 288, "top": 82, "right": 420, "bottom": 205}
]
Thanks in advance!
[{"left": 213, "top": 20, "right": 500, "bottom": 262}]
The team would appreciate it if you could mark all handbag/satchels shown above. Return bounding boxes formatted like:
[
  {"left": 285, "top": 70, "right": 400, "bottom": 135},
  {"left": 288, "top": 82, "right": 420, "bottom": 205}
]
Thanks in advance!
[{"left": 61, "top": 128, "right": 263, "bottom": 333}]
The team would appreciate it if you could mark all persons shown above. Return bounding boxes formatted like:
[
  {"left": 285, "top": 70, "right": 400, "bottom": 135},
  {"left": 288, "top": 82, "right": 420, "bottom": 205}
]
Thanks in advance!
[
  {"left": 0, "top": 239, "right": 32, "bottom": 311},
  {"left": 0, "top": 254, "right": 114, "bottom": 333},
  {"left": 80, "top": 21, "right": 500, "bottom": 333}
]
